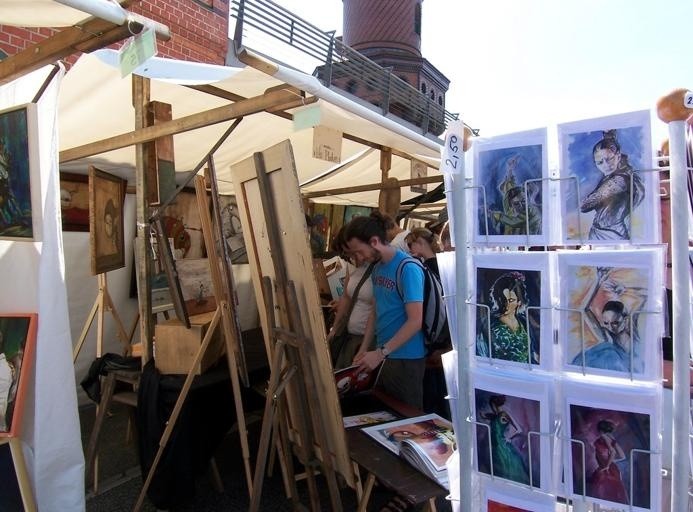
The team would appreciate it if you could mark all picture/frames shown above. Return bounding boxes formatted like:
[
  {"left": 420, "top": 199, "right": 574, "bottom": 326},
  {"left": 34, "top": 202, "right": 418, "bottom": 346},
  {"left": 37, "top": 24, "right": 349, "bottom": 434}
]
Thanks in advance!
[
  {"left": 0, "top": 102, "right": 42, "bottom": 244},
  {"left": 88, "top": 164, "right": 125, "bottom": 276},
  {"left": 129, "top": 185, "right": 212, "bottom": 299},
  {"left": 0, "top": 312, "right": 39, "bottom": 437},
  {"left": 60, "top": 171, "right": 88, "bottom": 232}
]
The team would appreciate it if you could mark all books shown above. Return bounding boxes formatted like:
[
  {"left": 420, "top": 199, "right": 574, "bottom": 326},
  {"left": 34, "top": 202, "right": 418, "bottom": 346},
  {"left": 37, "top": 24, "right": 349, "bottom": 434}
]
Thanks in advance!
[{"left": 360, "top": 413, "right": 456, "bottom": 491}]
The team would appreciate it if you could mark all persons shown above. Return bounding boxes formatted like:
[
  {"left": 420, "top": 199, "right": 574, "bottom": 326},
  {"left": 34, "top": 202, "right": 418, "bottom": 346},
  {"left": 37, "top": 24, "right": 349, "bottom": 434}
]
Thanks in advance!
[
  {"left": 577, "top": 127, "right": 645, "bottom": 242},
  {"left": 586, "top": 417, "right": 630, "bottom": 504},
  {"left": 326, "top": 210, "right": 454, "bottom": 418},
  {"left": 486, "top": 157, "right": 542, "bottom": 235},
  {"left": 473, "top": 271, "right": 539, "bottom": 364},
  {"left": 476, "top": 393, "right": 529, "bottom": 485},
  {"left": 568, "top": 267, "right": 648, "bottom": 375},
  {"left": 655, "top": 150, "right": 693, "bottom": 512}
]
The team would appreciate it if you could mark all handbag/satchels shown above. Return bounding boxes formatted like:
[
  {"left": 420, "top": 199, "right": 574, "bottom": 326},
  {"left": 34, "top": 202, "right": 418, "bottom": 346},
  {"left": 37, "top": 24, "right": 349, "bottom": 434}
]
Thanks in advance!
[{"left": 328, "top": 314, "right": 348, "bottom": 369}]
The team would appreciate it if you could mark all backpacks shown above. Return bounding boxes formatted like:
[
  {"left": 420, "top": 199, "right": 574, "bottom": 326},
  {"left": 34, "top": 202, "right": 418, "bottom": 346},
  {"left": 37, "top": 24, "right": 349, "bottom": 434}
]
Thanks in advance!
[{"left": 396, "top": 257, "right": 453, "bottom": 355}]
